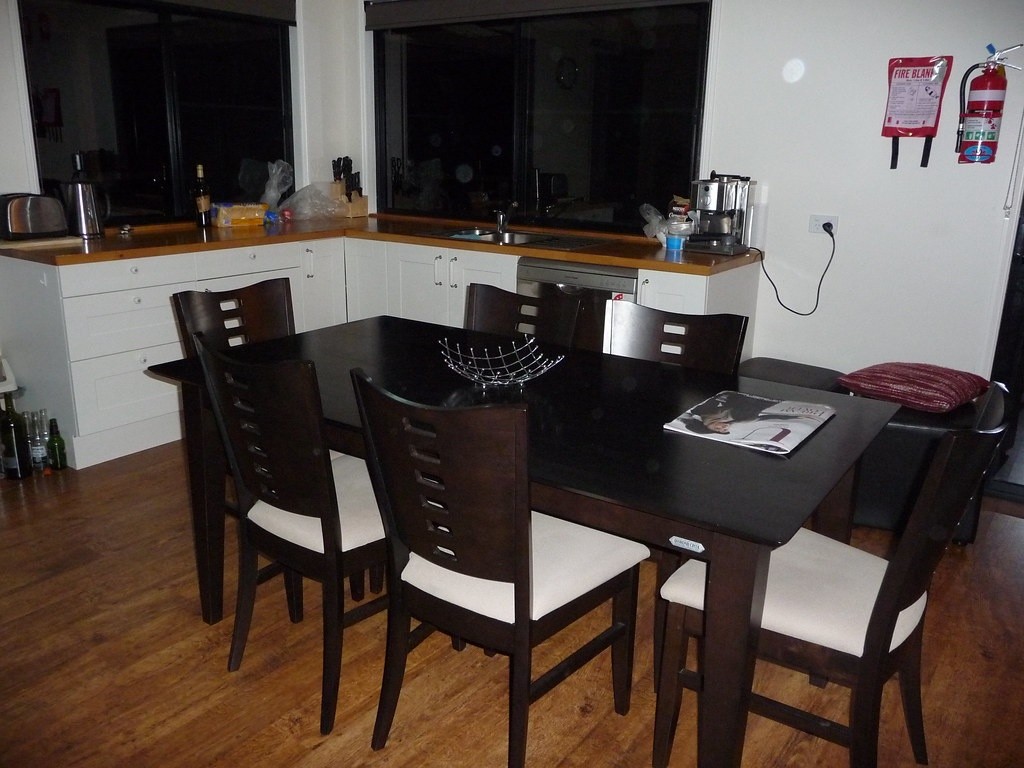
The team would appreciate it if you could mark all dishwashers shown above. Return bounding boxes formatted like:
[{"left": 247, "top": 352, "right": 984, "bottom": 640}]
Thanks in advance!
[{"left": 516, "top": 256, "right": 637, "bottom": 354}]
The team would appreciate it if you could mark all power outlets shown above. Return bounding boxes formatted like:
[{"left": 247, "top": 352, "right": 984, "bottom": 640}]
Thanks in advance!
[{"left": 808, "top": 215, "right": 838, "bottom": 233}]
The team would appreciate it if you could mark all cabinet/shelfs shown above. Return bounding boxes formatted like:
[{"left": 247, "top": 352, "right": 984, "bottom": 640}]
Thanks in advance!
[
  {"left": 345, "top": 237, "right": 387, "bottom": 324},
  {"left": 387, "top": 242, "right": 521, "bottom": 329},
  {"left": 0, "top": 250, "right": 199, "bottom": 470},
  {"left": 196, "top": 240, "right": 303, "bottom": 347},
  {"left": 299, "top": 237, "right": 348, "bottom": 333},
  {"left": 636, "top": 262, "right": 760, "bottom": 362}
]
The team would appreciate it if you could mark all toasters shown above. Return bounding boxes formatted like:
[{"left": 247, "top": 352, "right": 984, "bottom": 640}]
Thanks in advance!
[{"left": 0, "top": 192, "right": 67, "bottom": 240}]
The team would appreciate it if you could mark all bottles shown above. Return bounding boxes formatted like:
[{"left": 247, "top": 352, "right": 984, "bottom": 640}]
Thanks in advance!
[
  {"left": 39, "top": 408, "right": 49, "bottom": 440},
  {"left": 30, "top": 412, "right": 47, "bottom": 468},
  {"left": 21, "top": 410, "right": 32, "bottom": 442},
  {"left": 193, "top": 165, "right": 213, "bottom": 228},
  {"left": 0, "top": 392, "right": 32, "bottom": 479},
  {"left": 70, "top": 150, "right": 109, "bottom": 240},
  {"left": 47, "top": 419, "right": 67, "bottom": 470}
]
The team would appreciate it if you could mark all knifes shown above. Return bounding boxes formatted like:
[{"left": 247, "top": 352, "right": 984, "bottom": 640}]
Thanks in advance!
[{"left": 332, "top": 156, "right": 363, "bottom": 201}]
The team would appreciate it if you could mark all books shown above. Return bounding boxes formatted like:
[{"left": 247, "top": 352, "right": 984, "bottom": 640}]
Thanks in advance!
[{"left": 663, "top": 390, "right": 836, "bottom": 453}]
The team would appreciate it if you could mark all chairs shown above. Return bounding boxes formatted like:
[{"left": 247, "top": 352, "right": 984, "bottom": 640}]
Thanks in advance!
[{"left": 168, "top": 281, "right": 1007, "bottom": 768}]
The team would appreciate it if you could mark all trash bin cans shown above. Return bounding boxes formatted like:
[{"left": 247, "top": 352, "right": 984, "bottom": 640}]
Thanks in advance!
[{"left": 0, "top": 358, "right": 18, "bottom": 411}]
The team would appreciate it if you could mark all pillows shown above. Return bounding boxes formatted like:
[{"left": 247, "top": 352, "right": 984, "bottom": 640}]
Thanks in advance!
[{"left": 838, "top": 362, "right": 991, "bottom": 414}]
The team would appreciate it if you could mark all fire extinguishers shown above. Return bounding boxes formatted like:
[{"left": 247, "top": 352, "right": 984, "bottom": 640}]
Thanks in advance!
[{"left": 955, "top": 44, "right": 1024, "bottom": 164}]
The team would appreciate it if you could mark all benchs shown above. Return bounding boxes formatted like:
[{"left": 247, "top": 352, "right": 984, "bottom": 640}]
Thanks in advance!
[{"left": 734, "top": 358, "right": 1007, "bottom": 550}]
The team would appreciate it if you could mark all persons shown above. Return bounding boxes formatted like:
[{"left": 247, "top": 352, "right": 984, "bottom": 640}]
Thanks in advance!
[{"left": 677, "top": 392, "right": 819, "bottom": 435}]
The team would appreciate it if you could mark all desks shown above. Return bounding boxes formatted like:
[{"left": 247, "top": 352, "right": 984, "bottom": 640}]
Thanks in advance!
[{"left": 148, "top": 315, "right": 903, "bottom": 768}]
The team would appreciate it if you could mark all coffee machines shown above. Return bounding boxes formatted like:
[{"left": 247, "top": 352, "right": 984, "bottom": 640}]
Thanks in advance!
[{"left": 682, "top": 170, "right": 751, "bottom": 255}]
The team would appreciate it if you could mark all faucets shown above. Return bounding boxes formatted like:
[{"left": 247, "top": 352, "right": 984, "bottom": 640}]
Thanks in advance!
[{"left": 493, "top": 201, "right": 519, "bottom": 233}]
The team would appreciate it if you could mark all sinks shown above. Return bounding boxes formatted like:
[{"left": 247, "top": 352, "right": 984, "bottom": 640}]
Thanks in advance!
[
  {"left": 429, "top": 226, "right": 499, "bottom": 240},
  {"left": 468, "top": 231, "right": 559, "bottom": 245}
]
